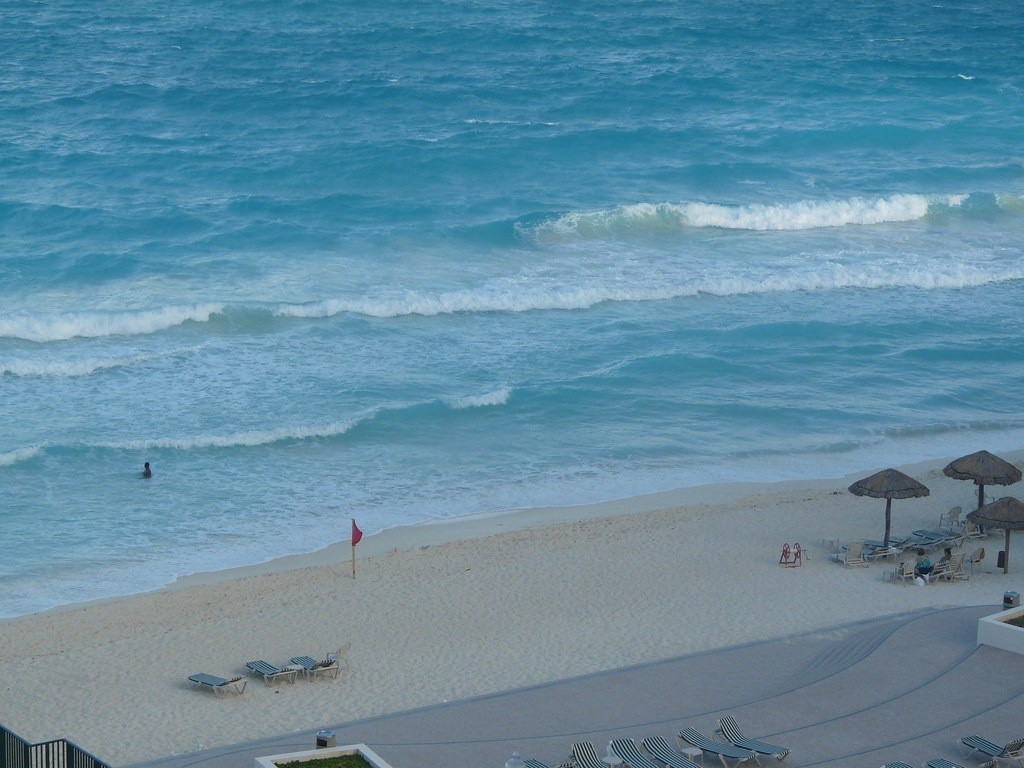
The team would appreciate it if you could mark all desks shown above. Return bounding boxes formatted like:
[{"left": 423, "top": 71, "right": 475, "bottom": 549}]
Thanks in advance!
[
  {"left": 602, "top": 756, "right": 622, "bottom": 768},
  {"left": 682, "top": 747, "right": 704, "bottom": 767}
]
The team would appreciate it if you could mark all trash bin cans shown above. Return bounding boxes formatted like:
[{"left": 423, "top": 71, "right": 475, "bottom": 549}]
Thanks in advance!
[
  {"left": 1003, "top": 591, "right": 1019, "bottom": 611},
  {"left": 316, "top": 731, "right": 336, "bottom": 749}
]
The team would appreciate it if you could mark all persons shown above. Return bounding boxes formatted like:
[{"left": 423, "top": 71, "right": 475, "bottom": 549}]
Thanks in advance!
[
  {"left": 144, "top": 462, "right": 151, "bottom": 478},
  {"left": 913, "top": 548, "right": 931, "bottom": 574},
  {"left": 940, "top": 547, "right": 952, "bottom": 562}
]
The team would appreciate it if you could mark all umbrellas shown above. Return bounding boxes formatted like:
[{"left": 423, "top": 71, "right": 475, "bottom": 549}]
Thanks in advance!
[
  {"left": 943, "top": 450, "right": 1022, "bottom": 534},
  {"left": 847, "top": 468, "right": 929, "bottom": 558},
  {"left": 965, "top": 496, "right": 1024, "bottom": 574}
]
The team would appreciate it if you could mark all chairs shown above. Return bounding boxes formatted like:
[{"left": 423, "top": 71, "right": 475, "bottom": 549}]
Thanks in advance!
[
  {"left": 607, "top": 738, "right": 659, "bottom": 768},
  {"left": 711, "top": 716, "right": 791, "bottom": 766},
  {"left": 246, "top": 660, "right": 297, "bottom": 688},
  {"left": 832, "top": 489, "right": 1004, "bottom": 584},
  {"left": 326, "top": 642, "right": 351, "bottom": 679},
  {"left": 523, "top": 758, "right": 577, "bottom": 768},
  {"left": 291, "top": 655, "right": 337, "bottom": 681},
  {"left": 922, "top": 757, "right": 966, "bottom": 768},
  {"left": 957, "top": 734, "right": 1024, "bottom": 768},
  {"left": 679, "top": 727, "right": 762, "bottom": 768},
  {"left": 187, "top": 672, "right": 247, "bottom": 699},
  {"left": 881, "top": 761, "right": 915, "bottom": 768},
  {"left": 572, "top": 741, "right": 607, "bottom": 768},
  {"left": 641, "top": 736, "right": 703, "bottom": 768}
]
